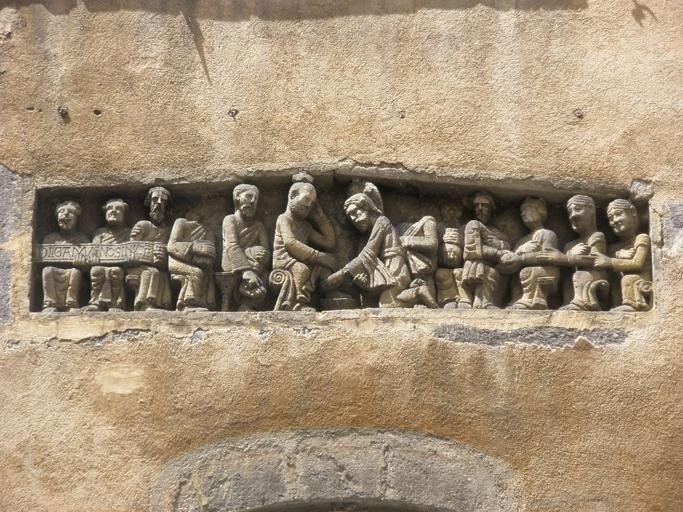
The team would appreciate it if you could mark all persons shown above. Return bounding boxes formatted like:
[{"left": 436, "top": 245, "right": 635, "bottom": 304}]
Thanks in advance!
[{"left": 39, "top": 172, "right": 653, "bottom": 311}]
